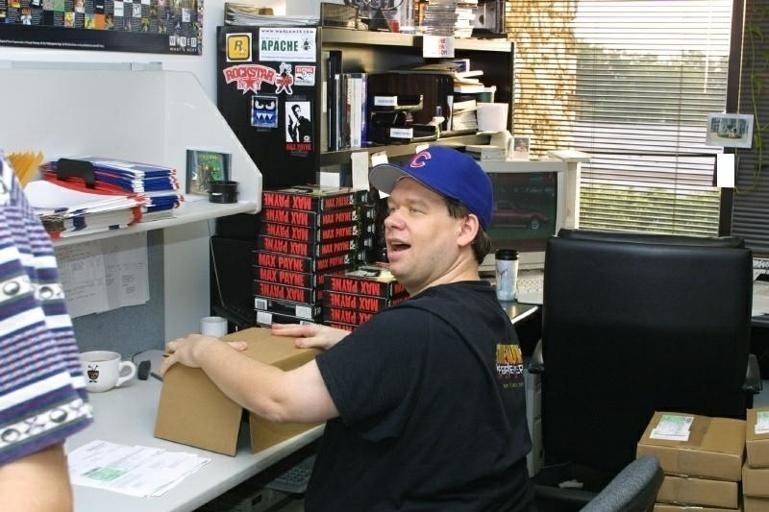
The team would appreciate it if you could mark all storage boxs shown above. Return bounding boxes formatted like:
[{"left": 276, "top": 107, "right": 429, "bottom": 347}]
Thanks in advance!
[{"left": 154, "top": 327, "right": 327, "bottom": 456}]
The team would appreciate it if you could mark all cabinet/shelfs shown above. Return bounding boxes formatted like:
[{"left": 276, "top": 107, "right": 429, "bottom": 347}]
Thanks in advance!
[{"left": 210, "top": 24, "right": 516, "bottom": 315}]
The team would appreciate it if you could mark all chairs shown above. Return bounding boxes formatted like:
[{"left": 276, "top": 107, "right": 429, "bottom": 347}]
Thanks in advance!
[
  {"left": 528, "top": 453, "right": 665, "bottom": 511},
  {"left": 527, "top": 227, "right": 763, "bottom": 489}
]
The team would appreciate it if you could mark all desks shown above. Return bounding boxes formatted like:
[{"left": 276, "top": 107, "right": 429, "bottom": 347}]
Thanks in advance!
[
  {"left": 480, "top": 266, "right": 768, "bottom": 474},
  {"left": 66, "top": 350, "right": 325, "bottom": 511}
]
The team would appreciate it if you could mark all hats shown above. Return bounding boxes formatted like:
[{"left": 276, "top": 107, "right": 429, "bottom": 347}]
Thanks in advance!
[{"left": 368, "top": 146, "right": 493, "bottom": 232}]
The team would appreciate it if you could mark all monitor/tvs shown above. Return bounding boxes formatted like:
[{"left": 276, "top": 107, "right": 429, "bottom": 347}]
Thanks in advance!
[{"left": 474, "top": 159, "right": 580, "bottom": 271}]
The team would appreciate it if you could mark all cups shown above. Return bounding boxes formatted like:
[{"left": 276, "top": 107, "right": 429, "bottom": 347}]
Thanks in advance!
[
  {"left": 77, "top": 349, "right": 137, "bottom": 393},
  {"left": 206, "top": 179, "right": 239, "bottom": 204},
  {"left": 476, "top": 102, "right": 511, "bottom": 133},
  {"left": 198, "top": 316, "right": 229, "bottom": 339},
  {"left": 494, "top": 247, "right": 520, "bottom": 301}
]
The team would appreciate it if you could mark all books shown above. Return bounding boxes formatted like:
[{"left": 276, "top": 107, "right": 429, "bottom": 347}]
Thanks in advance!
[{"left": 320, "top": 0, "right": 519, "bottom": 155}]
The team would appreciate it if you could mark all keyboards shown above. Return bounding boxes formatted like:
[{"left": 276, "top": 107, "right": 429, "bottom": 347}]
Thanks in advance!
[{"left": 516, "top": 276, "right": 543, "bottom": 305}]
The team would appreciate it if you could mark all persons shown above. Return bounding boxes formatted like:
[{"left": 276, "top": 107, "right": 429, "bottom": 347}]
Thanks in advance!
[
  {"left": 158, "top": 147, "right": 534, "bottom": 511},
  {"left": 0, "top": 158, "right": 97, "bottom": 511},
  {"left": 288, "top": 103, "right": 310, "bottom": 143}
]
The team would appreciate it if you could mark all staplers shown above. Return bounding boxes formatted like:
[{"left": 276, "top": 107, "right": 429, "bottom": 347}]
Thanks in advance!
[{"left": 366, "top": 112, "right": 410, "bottom": 146}]
